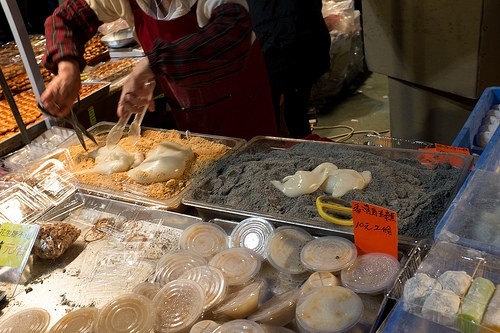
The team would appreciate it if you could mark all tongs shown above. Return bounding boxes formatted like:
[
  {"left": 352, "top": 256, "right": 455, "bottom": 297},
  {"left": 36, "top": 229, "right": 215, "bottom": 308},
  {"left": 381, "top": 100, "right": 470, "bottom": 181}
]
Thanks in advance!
[{"left": 105, "top": 80, "right": 156, "bottom": 152}]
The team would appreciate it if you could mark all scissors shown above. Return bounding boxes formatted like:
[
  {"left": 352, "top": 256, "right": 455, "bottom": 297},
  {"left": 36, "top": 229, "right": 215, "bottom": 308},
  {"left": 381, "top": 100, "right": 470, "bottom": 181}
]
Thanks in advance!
[
  {"left": 36, "top": 93, "right": 99, "bottom": 152},
  {"left": 316, "top": 192, "right": 358, "bottom": 229}
]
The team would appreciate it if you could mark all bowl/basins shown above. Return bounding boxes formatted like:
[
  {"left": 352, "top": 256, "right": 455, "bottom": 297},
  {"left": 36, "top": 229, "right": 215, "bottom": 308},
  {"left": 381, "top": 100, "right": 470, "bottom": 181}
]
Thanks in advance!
[
  {"left": 99, "top": 30, "right": 134, "bottom": 48},
  {"left": 0, "top": 216, "right": 400, "bottom": 333}
]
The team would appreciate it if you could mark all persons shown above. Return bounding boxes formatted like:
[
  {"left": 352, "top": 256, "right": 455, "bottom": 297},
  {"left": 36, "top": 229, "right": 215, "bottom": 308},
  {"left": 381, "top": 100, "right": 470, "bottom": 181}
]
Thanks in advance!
[
  {"left": 246, "top": 0, "right": 331, "bottom": 140},
  {"left": 39, "top": 0, "right": 279, "bottom": 142}
]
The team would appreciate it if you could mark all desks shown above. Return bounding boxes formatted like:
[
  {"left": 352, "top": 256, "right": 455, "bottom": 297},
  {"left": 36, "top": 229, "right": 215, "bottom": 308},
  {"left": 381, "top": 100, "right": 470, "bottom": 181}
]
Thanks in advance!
[{"left": 0, "top": 32, "right": 149, "bottom": 158}]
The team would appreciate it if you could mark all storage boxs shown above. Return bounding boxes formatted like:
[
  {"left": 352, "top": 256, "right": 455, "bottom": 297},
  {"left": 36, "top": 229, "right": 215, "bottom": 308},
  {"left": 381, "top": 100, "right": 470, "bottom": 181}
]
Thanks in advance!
[
  {"left": 360, "top": 0, "right": 500, "bottom": 101},
  {"left": 386, "top": 77, "right": 473, "bottom": 148},
  {"left": 0, "top": 87, "right": 500, "bottom": 333}
]
